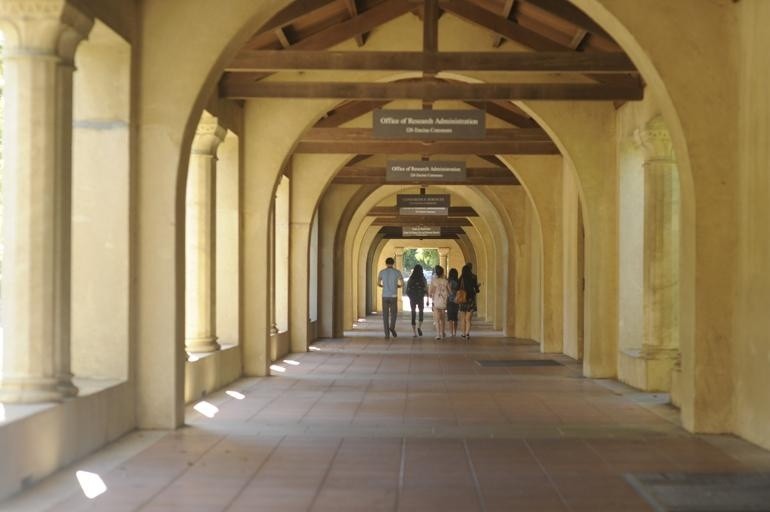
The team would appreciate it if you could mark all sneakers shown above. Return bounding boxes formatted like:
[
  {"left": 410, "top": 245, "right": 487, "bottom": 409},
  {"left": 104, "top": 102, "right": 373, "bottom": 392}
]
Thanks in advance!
[
  {"left": 389, "top": 327, "right": 397, "bottom": 337},
  {"left": 417, "top": 328, "right": 422, "bottom": 336},
  {"left": 434, "top": 334, "right": 470, "bottom": 340}
]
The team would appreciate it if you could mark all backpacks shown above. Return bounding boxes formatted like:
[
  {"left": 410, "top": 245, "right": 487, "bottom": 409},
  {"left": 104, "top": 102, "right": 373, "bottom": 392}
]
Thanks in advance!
[{"left": 433, "top": 280, "right": 448, "bottom": 310}]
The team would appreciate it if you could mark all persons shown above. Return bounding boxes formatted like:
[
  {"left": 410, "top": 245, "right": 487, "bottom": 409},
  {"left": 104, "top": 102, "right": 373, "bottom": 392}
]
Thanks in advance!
[{"left": 377, "top": 257, "right": 482, "bottom": 339}]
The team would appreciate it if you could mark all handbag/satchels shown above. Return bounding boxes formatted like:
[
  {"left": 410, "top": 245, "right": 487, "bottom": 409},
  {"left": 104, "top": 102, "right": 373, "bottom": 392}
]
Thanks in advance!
[{"left": 453, "top": 277, "right": 468, "bottom": 304}]
